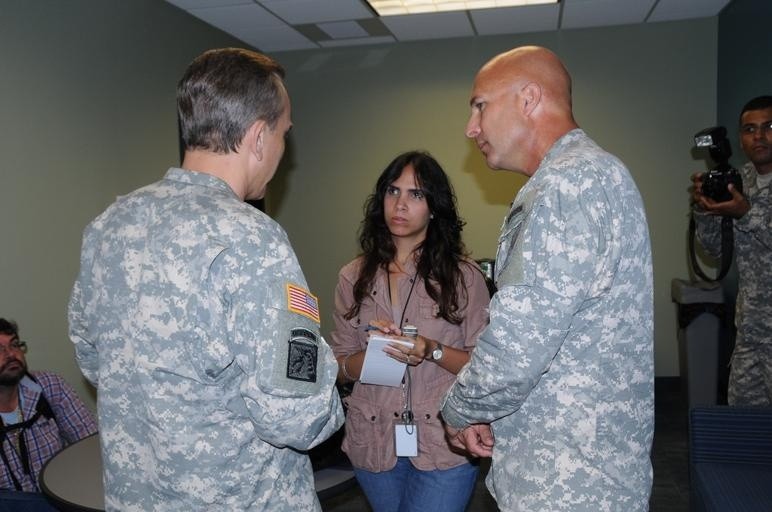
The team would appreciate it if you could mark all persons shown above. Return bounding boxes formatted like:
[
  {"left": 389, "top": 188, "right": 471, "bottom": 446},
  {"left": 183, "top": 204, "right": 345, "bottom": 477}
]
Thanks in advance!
[
  {"left": 330, "top": 150, "right": 491, "bottom": 512},
  {"left": 0, "top": 317, "right": 99, "bottom": 512},
  {"left": 689, "top": 95, "right": 772, "bottom": 407},
  {"left": 69, "top": 44, "right": 346, "bottom": 512},
  {"left": 437, "top": 43, "right": 655, "bottom": 512}
]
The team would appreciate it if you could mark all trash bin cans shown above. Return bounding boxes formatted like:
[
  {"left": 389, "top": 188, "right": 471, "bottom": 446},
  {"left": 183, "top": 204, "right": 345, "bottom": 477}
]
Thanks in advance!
[{"left": 671, "top": 278, "right": 735, "bottom": 420}]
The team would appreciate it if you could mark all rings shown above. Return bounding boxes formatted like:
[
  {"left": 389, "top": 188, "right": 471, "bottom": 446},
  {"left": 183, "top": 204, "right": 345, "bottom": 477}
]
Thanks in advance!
[
  {"left": 409, "top": 343, "right": 417, "bottom": 350},
  {"left": 406, "top": 354, "right": 410, "bottom": 362}
]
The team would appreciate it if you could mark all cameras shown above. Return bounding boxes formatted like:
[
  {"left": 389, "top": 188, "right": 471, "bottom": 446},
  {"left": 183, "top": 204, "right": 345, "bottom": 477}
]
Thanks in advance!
[{"left": 694, "top": 126, "right": 743, "bottom": 203}]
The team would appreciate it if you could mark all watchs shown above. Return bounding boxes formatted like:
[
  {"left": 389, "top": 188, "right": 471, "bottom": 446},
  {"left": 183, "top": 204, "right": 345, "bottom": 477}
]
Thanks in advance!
[{"left": 425, "top": 340, "right": 442, "bottom": 361}]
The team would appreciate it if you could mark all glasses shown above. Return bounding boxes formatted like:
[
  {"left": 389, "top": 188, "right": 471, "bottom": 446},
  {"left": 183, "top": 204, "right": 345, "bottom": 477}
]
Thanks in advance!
[{"left": 0, "top": 340, "right": 29, "bottom": 354}]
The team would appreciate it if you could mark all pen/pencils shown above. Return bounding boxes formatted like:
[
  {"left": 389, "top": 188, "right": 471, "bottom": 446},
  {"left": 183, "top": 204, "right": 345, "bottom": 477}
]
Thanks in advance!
[{"left": 367, "top": 326, "right": 376, "bottom": 330}]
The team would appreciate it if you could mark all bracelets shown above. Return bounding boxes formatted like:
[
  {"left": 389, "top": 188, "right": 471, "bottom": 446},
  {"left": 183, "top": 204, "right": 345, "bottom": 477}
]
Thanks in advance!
[{"left": 342, "top": 351, "right": 358, "bottom": 380}]
